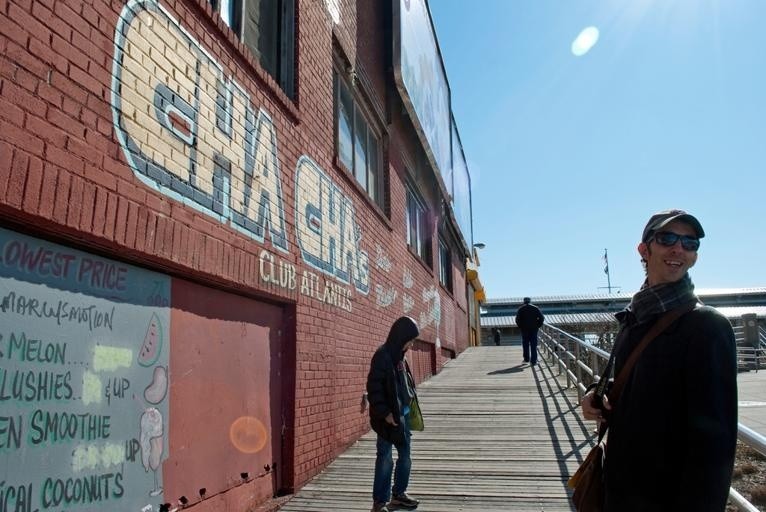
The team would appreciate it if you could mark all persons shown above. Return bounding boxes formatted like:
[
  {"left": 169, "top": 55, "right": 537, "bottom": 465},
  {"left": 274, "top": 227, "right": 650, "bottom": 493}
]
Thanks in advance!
[
  {"left": 515, "top": 297, "right": 545, "bottom": 366},
  {"left": 580, "top": 208, "right": 741, "bottom": 512},
  {"left": 363, "top": 316, "right": 424, "bottom": 512},
  {"left": 493, "top": 326, "right": 501, "bottom": 346}
]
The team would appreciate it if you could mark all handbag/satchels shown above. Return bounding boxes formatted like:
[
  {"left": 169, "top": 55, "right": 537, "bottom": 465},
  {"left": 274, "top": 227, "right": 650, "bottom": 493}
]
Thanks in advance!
[
  {"left": 408, "top": 397, "right": 425, "bottom": 434},
  {"left": 568, "top": 446, "right": 605, "bottom": 512}
]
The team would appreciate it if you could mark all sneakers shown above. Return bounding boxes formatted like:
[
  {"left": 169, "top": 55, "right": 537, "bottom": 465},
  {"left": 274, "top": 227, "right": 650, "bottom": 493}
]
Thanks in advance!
[
  {"left": 391, "top": 492, "right": 420, "bottom": 507},
  {"left": 373, "top": 502, "right": 390, "bottom": 512}
]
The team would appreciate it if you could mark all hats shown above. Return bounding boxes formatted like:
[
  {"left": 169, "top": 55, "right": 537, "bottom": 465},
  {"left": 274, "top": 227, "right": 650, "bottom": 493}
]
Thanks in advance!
[{"left": 641, "top": 208, "right": 706, "bottom": 243}]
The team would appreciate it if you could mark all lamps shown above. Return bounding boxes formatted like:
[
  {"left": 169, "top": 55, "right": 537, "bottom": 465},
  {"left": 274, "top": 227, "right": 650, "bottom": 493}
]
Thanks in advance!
[{"left": 473, "top": 243, "right": 486, "bottom": 249}]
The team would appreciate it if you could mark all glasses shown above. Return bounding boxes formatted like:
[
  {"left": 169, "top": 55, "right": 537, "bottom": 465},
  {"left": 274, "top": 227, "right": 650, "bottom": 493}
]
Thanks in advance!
[{"left": 647, "top": 230, "right": 700, "bottom": 251}]
